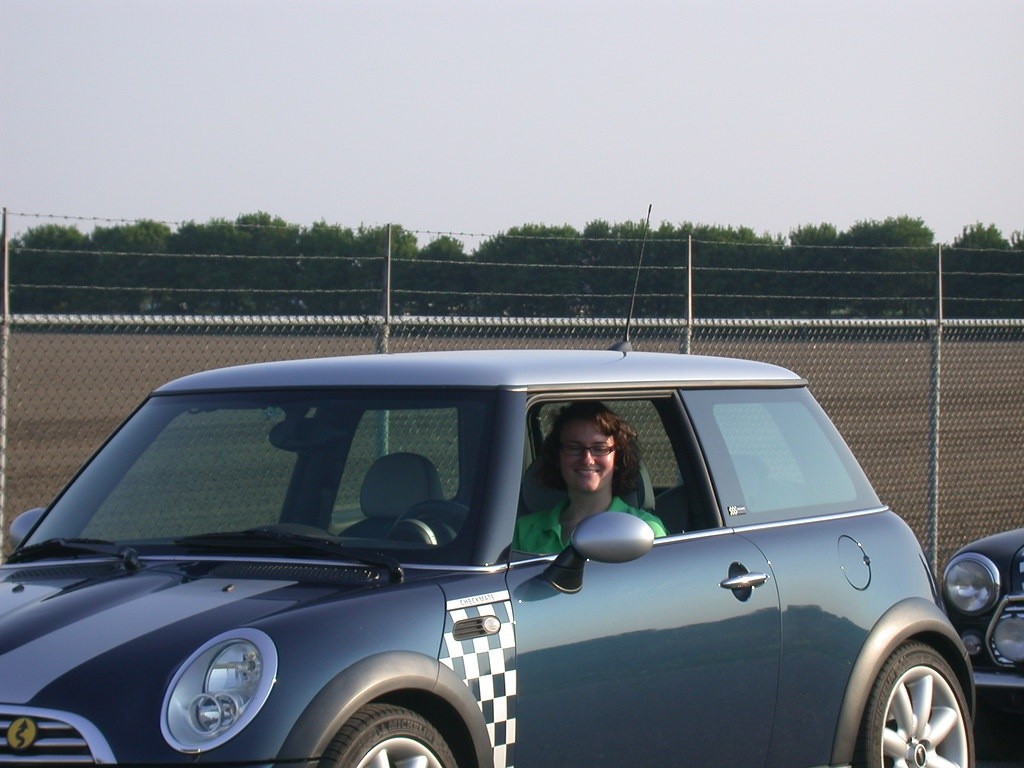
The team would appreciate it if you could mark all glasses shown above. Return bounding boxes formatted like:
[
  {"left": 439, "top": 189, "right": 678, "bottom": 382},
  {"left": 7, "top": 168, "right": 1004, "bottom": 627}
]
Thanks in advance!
[{"left": 558, "top": 443, "right": 617, "bottom": 456}]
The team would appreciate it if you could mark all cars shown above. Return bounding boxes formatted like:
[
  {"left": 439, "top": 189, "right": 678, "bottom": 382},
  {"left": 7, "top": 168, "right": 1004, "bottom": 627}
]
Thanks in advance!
[
  {"left": 939, "top": 526, "right": 1024, "bottom": 751},
  {"left": 0, "top": 350, "right": 977, "bottom": 768}
]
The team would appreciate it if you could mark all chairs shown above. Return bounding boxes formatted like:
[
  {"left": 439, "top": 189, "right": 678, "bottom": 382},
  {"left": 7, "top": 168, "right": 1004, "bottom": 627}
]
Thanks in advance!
[
  {"left": 337, "top": 453, "right": 443, "bottom": 540},
  {"left": 522, "top": 451, "right": 656, "bottom": 512}
]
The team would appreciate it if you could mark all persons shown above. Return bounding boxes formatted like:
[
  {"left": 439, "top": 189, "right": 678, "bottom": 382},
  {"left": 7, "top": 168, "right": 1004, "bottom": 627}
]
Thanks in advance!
[{"left": 512, "top": 401, "right": 667, "bottom": 555}]
